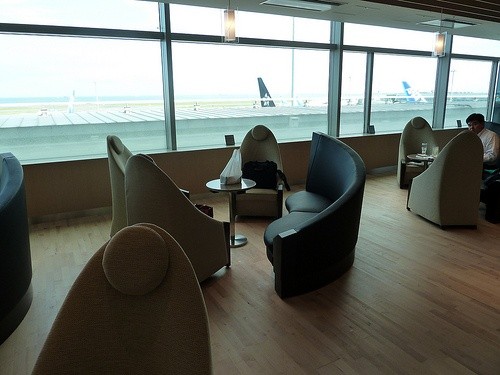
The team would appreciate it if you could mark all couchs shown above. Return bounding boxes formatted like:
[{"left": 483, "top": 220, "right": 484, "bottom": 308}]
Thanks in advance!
[
  {"left": 475, "top": 122, "right": 500, "bottom": 224},
  {"left": 267, "top": 131, "right": 366, "bottom": 301},
  {"left": 29, "top": 223, "right": 212, "bottom": 375},
  {"left": 397, "top": 116, "right": 440, "bottom": 189},
  {"left": 106, "top": 134, "right": 230, "bottom": 285},
  {"left": 228, "top": 124, "right": 285, "bottom": 219},
  {"left": 0, "top": 153, "right": 32, "bottom": 346},
  {"left": 406, "top": 130, "right": 484, "bottom": 230}
]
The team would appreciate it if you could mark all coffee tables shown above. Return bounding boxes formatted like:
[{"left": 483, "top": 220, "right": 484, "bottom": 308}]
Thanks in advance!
[
  {"left": 407, "top": 154, "right": 437, "bottom": 171},
  {"left": 206, "top": 179, "right": 256, "bottom": 248}
]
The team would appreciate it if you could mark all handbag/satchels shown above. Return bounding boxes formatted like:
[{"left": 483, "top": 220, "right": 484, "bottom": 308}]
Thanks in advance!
[
  {"left": 220, "top": 149, "right": 242, "bottom": 186},
  {"left": 243, "top": 160, "right": 291, "bottom": 191}
]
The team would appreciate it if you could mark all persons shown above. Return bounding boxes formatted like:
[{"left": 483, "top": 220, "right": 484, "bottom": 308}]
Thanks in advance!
[{"left": 466, "top": 113, "right": 500, "bottom": 163}]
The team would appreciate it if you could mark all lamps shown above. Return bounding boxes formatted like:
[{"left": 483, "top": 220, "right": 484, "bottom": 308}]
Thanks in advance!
[
  {"left": 220, "top": 0, "right": 239, "bottom": 46},
  {"left": 430, "top": 9, "right": 446, "bottom": 58}
]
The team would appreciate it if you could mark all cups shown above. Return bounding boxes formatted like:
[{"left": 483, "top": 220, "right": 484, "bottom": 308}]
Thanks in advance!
[
  {"left": 433, "top": 147, "right": 439, "bottom": 158},
  {"left": 422, "top": 143, "right": 427, "bottom": 155}
]
